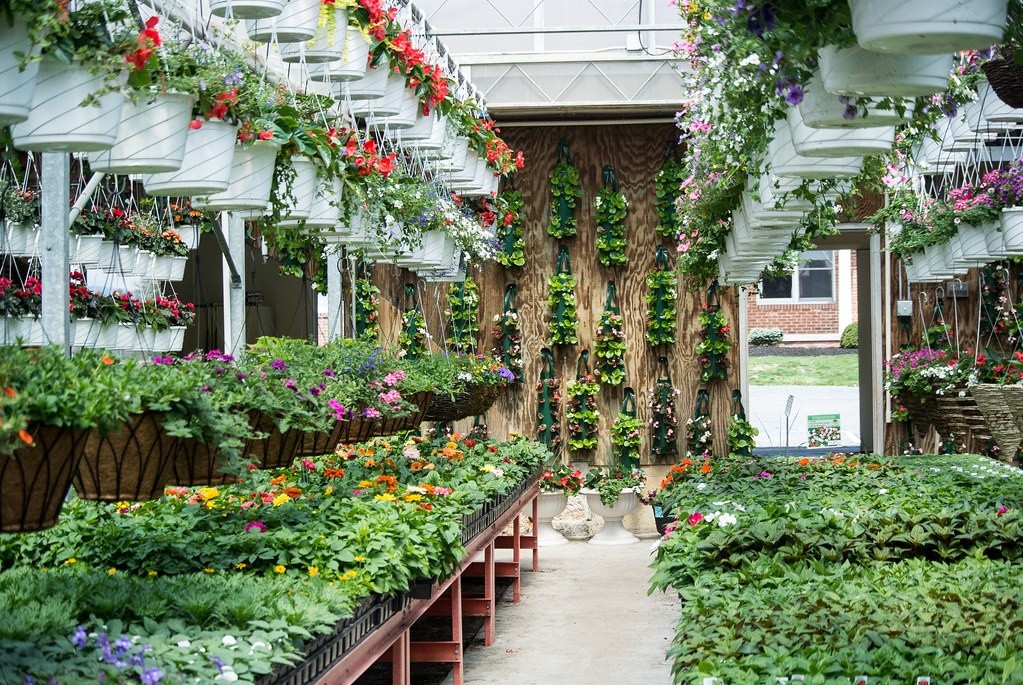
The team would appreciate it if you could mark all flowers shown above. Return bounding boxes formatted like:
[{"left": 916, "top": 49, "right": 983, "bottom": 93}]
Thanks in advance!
[{"left": 0, "top": 0, "right": 1023, "bottom": 684}]
